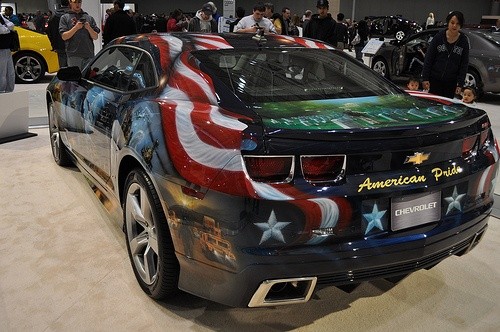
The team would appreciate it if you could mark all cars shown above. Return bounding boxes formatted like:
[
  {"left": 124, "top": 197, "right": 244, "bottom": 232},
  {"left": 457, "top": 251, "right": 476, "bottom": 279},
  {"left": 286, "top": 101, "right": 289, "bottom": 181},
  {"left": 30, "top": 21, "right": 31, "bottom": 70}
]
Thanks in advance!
[
  {"left": 7, "top": 23, "right": 60, "bottom": 85},
  {"left": 45, "top": 31, "right": 500, "bottom": 311},
  {"left": 371, "top": 27, "right": 500, "bottom": 104}
]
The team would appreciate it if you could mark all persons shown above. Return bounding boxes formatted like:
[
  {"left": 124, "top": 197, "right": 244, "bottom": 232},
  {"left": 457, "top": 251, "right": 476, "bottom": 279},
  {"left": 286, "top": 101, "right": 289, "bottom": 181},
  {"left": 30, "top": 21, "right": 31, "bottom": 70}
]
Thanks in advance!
[
  {"left": 425, "top": 12, "right": 435, "bottom": 30},
  {"left": 0, "top": 0, "right": 382, "bottom": 93},
  {"left": 406, "top": 78, "right": 430, "bottom": 94},
  {"left": 418, "top": 10, "right": 469, "bottom": 103},
  {"left": 458, "top": 86, "right": 478, "bottom": 109}
]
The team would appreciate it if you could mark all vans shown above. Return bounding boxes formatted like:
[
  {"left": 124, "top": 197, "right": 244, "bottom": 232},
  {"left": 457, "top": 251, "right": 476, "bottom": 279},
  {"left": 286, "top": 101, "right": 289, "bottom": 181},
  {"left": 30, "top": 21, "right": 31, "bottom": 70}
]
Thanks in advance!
[{"left": 364, "top": 14, "right": 423, "bottom": 40}]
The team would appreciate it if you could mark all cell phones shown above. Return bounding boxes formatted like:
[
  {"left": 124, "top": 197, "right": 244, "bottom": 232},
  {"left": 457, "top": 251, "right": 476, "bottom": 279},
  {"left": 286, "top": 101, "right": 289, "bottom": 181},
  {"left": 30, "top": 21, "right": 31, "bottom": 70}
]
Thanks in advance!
[{"left": 80, "top": 17, "right": 86, "bottom": 24}]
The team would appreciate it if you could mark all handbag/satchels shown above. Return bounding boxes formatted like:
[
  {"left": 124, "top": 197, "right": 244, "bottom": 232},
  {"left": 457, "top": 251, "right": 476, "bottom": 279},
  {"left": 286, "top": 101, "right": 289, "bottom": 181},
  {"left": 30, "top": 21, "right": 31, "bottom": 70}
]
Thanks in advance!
[{"left": 351, "top": 33, "right": 360, "bottom": 45}]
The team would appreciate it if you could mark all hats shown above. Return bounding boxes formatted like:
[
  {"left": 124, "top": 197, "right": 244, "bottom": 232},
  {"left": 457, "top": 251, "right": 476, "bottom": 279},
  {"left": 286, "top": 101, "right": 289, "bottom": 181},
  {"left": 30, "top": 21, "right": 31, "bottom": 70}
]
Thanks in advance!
[
  {"left": 315, "top": 0, "right": 329, "bottom": 8},
  {"left": 305, "top": 10, "right": 312, "bottom": 13},
  {"left": 201, "top": 4, "right": 213, "bottom": 15}
]
